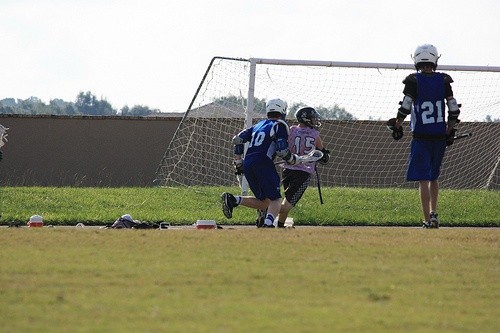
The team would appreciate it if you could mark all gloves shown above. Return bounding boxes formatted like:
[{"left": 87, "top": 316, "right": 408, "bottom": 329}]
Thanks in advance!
[
  {"left": 387, "top": 118, "right": 403, "bottom": 140},
  {"left": 446, "top": 130, "right": 458, "bottom": 146},
  {"left": 234, "top": 159, "right": 243, "bottom": 176},
  {"left": 287, "top": 152, "right": 302, "bottom": 166},
  {"left": 317, "top": 148, "right": 330, "bottom": 165}
]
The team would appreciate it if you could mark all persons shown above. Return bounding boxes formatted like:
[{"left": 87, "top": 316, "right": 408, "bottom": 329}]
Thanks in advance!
[
  {"left": 220, "top": 99, "right": 304, "bottom": 229},
  {"left": 257, "top": 107, "right": 331, "bottom": 229},
  {"left": 386, "top": 44, "right": 461, "bottom": 230}
]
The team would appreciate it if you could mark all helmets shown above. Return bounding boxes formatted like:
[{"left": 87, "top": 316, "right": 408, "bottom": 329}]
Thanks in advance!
[
  {"left": 266, "top": 98, "right": 285, "bottom": 116},
  {"left": 411, "top": 44, "right": 441, "bottom": 71},
  {"left": 295, "top": 107, "right": 321, "bottom": 130}
]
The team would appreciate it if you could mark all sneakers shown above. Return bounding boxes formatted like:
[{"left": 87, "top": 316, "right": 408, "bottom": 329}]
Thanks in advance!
[
  {"left": 256, "top": 208, "right": 265, "bottom": 228},
  {"left": 220, "top": 192, "right": 238, "bottom": 219},
  {"left": 277, "top": 226, "right": 286, "bottom": 228},
  {"left": 423, "top": 212, "right": 439, "bottom": 228},
  {"left": 262, "top": 224, "right": 275, "bottom": 228}
]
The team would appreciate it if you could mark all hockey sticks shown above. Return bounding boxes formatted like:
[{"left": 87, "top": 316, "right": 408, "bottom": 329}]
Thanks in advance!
[
  {"left": 453, "top": 131, "right": 472, "bottom": 140},
  {"left": 314, "top": 164, "right": 324, "bottom": 204},
  {"left": 274, "top": 149, "right": 324, "bottom": 166}
]
